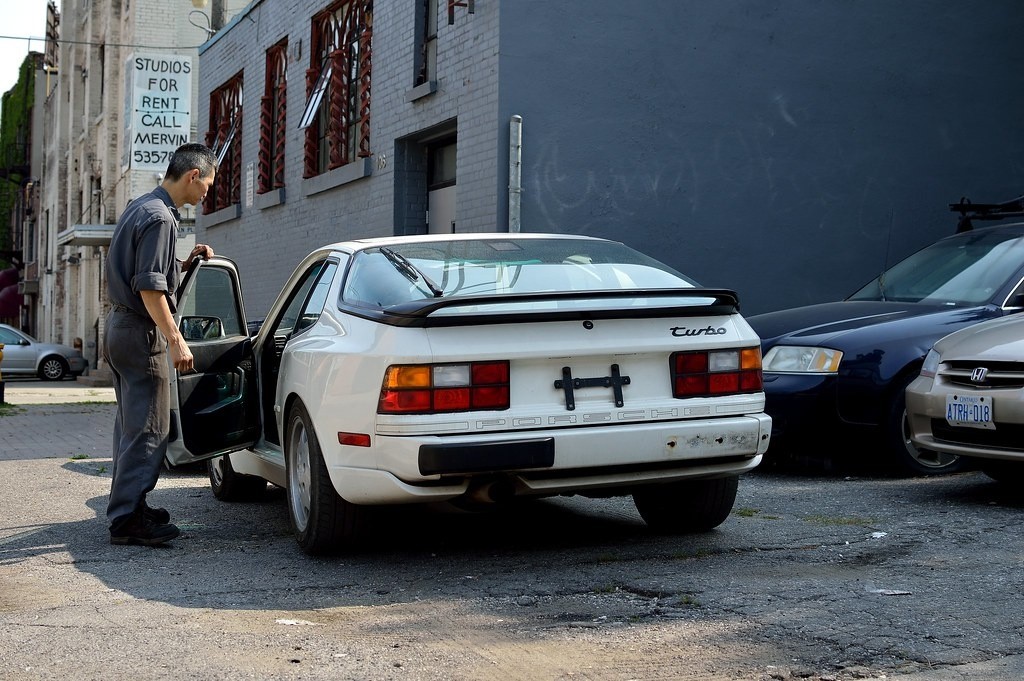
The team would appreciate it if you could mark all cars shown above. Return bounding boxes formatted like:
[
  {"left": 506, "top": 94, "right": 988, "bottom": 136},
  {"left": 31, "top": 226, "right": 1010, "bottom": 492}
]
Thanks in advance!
[
  {"left": 165, "top": 231, "right": 775, "bottom": 560},
  {"left": 0, "top": 322, "right": 88, "bottom": 381},
  {"left": 903, "top": 272, "right": 1024, "bottom": 510},
  {"left": 744, "top": 223, "right": 1024, "bottom": 469}
]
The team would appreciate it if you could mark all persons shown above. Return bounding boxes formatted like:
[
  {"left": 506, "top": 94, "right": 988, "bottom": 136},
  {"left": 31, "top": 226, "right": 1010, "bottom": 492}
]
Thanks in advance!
[{"left": 103, "top": 143, "right": 219, "bottom": 545}]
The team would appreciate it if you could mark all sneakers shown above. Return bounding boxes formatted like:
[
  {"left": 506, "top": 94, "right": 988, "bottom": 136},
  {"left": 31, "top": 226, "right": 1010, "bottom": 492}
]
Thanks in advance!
[
  {"left": 109, "top": 511, "right": 180, "bottom": 545},
  {"left": 144, "top": 502, "right": 170, "bottom": 524}
]
